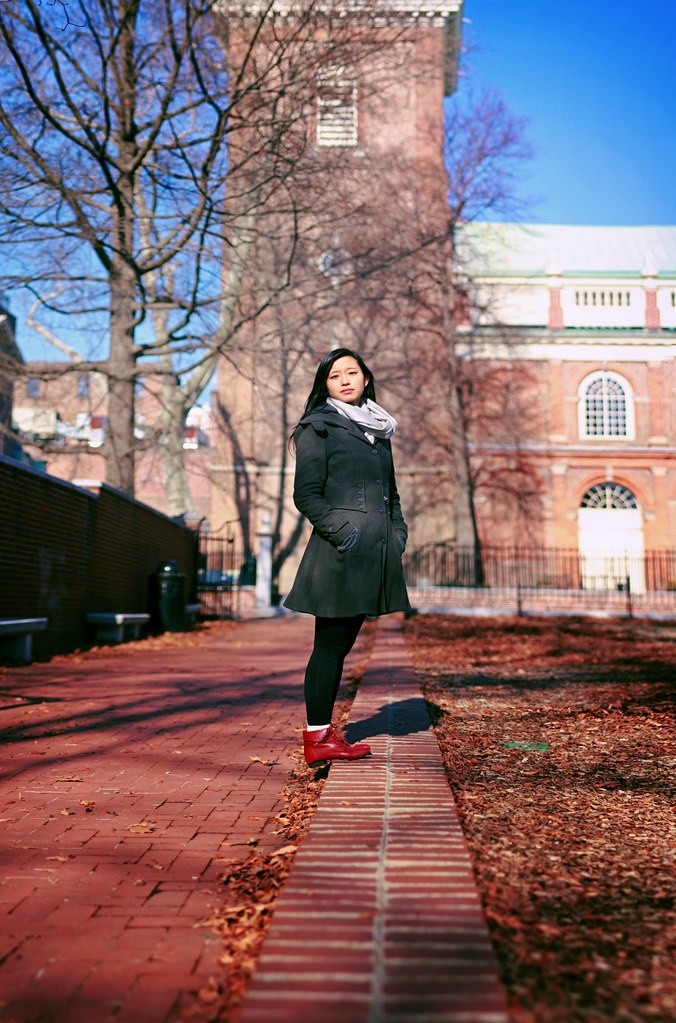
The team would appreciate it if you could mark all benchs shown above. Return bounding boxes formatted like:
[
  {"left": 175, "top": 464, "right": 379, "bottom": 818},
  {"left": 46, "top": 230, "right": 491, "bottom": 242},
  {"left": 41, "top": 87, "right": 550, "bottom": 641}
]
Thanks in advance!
[
  {"left": 186, "top": 603, "right": 203, "bottom": 621},
  {"left": 89, "top": 613, "right": 150, "bottom": 642},
  {"left": 0, "top": 616, "right": 48, "bottom": 662}
]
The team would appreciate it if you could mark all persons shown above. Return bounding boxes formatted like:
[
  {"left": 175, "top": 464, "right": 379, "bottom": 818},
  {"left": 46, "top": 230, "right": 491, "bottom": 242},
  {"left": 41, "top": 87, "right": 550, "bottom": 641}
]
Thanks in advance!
[{"left": 283, "top": 347, "right": 411, "bottom": 767}]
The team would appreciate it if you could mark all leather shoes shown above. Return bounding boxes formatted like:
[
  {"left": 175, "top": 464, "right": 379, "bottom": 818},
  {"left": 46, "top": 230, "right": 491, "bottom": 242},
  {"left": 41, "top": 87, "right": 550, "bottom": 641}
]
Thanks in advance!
[{"left": 303, "top": 723, "right": 370, "bottom": 768}]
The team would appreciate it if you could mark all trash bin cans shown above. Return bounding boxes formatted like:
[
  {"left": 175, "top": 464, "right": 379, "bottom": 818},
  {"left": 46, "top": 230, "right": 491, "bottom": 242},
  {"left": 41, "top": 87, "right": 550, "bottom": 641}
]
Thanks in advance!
[{"left": 150, "top": 562, "right": 187, "bottom": 636}]
